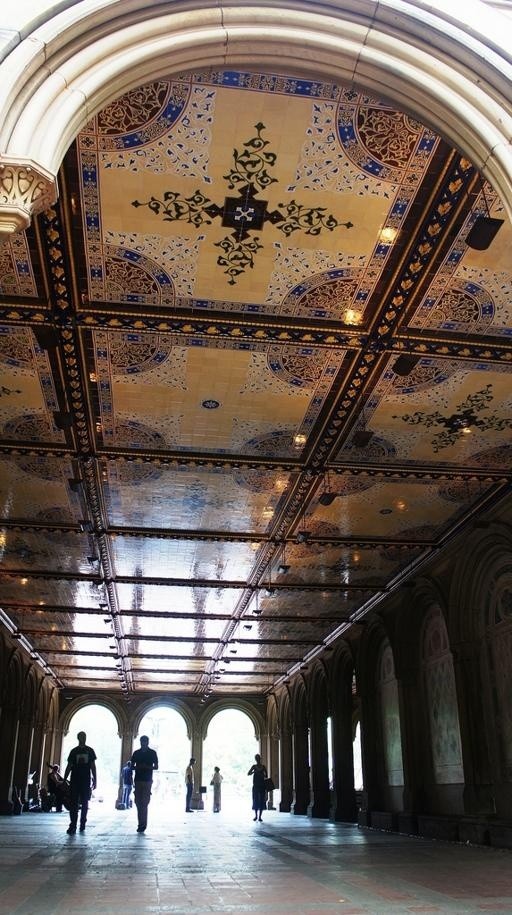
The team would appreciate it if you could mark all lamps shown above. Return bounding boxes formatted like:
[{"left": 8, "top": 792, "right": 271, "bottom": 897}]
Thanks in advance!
[
  {"left": 9, "top": 280, "right": 133, "bottom": 707},
  {"left": 251, "top": 584, "right": 263, "bottom": 615},
  {"left": 264, "top": 561, "right": 280, "bottom": 599},
  {"left": 277, "top": 540, "right": 292, "bottom": 575},
  {"left": 319, "top": 471, "right": 337, "bottom": 506},
  {"left": 453, "top": 156, "right": 508, "bottom": 254},
  {"left": 297, "top": 514, "right": 311, "bottom": 544},
  {"left": 199, "top": 605, "right": 253, "bottom": 708},
  {"left": 389, "top": 305, "right": 421, "bottom": 380}
]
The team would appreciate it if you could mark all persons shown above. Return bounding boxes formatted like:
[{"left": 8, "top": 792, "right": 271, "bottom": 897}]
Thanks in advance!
[
  {"left": 121, "top": 761, "right": 133, "bottom": 809},
  {"left": 61, "top": 732, "right": 97, "bottom": 833},
  {"left": 47, "top": 764, "right": 64, "bottom": 812},
  {"left": 129, "top": 736, "right": 158, "bottom": 832},
  {"left": 247, "top": 754, "right": 268, "bottom": 821},
  {"left": 185, "top": 758, "right": 196, "bottom": 812},
  {"left": 211, "top": 767, "right": 223, "bottom": 813}
]
